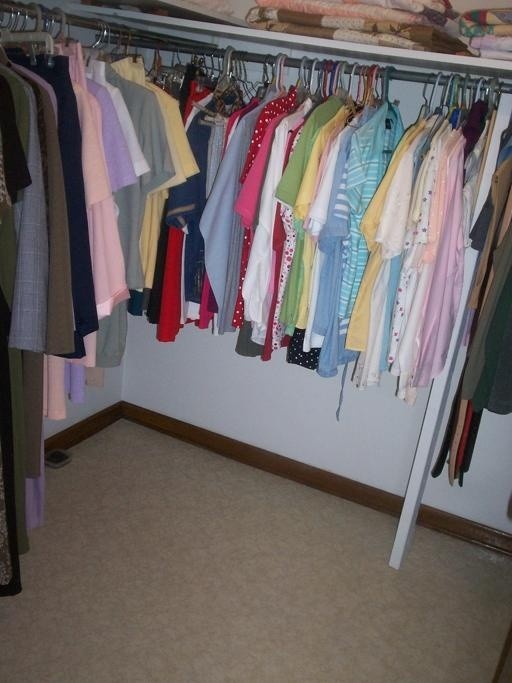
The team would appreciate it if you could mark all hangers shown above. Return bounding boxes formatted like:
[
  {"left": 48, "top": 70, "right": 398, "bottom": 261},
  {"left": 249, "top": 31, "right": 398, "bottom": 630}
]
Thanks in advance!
[
  {"left": 92, "top": 31, "right": 512, "bottom": 152},
  {"left": 0, "top": 0, "right": 165, "bottom": 90}
]
